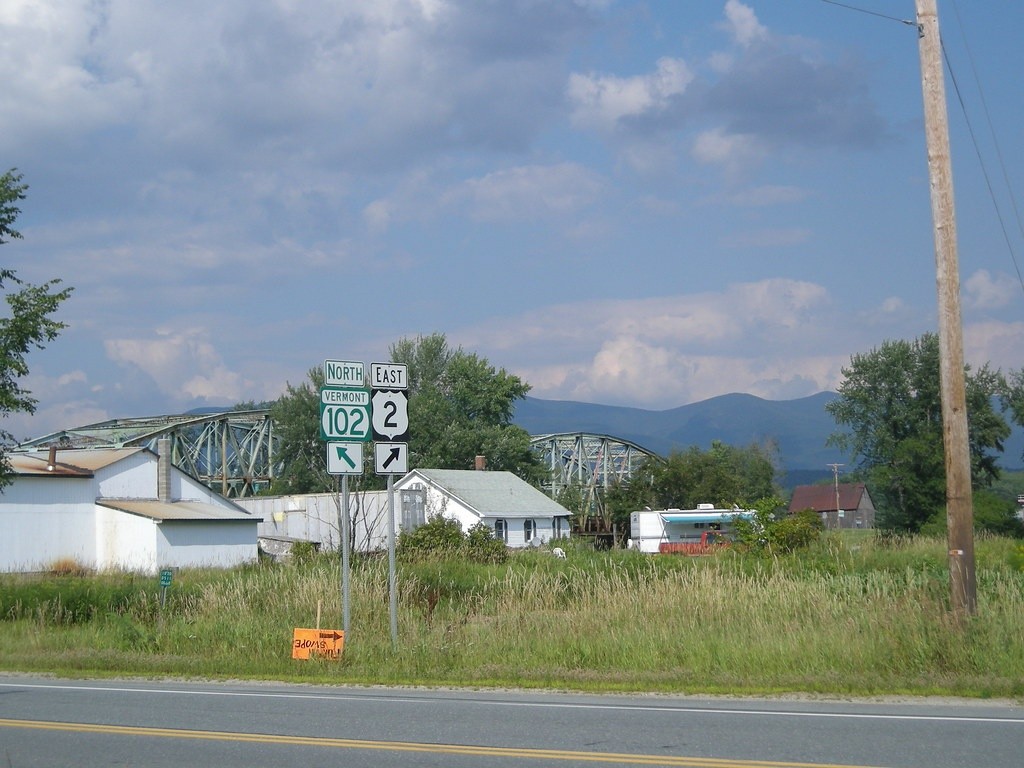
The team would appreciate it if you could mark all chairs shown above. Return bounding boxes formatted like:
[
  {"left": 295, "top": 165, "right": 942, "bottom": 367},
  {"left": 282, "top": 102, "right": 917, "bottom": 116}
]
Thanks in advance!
[{"left": 553, "top": 547, "right": 566, "bottom": 561}]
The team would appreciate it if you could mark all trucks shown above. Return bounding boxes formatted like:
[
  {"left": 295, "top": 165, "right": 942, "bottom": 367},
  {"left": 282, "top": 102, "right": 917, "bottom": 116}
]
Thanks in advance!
[{"left": 658, "top": 529, "right": 732, "bottom": 557}]
